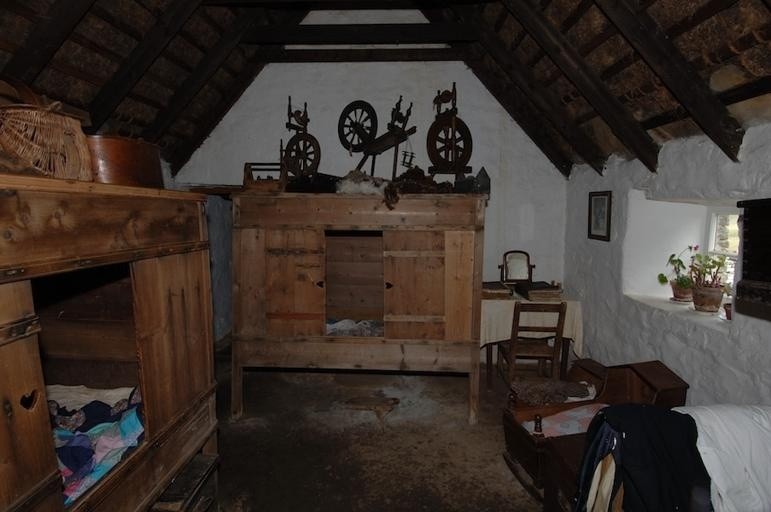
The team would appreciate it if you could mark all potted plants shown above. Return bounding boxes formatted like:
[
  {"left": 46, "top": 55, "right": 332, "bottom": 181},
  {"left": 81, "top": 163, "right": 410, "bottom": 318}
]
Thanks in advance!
[{"left": 659, "top": 243, "right": 732, "bottom": 313}]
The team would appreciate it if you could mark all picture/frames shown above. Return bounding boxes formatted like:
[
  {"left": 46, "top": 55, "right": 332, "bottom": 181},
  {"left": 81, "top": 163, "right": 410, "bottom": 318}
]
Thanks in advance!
[{"left": 585, "top": 189, "right": 612, "bottom": 243}]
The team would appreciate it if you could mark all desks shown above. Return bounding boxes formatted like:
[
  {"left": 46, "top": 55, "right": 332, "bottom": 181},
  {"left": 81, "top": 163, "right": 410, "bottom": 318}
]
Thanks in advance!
[{"left": 480, "top": 297, "right": 584, "bottom": 369}]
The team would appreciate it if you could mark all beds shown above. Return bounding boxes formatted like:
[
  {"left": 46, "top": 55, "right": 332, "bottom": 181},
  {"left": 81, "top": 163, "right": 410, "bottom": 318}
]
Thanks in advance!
[
  {"left": 0, "top": 175, "right": 220, "bottom": 512},
  {"left": 498, "top": 360, "right": 690, "bottom": 512},
  {"left": 232, "top": 189, "right": 489, "bottom": 426}
]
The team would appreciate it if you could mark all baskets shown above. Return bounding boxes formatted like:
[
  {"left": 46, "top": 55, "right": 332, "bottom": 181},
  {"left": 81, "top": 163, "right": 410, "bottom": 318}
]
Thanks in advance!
[{"left": 0, "top": 107, "right": 93, "bottom": 182}]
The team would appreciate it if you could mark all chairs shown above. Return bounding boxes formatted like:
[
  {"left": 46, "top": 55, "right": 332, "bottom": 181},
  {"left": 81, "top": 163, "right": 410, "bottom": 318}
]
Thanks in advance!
[{"left": 497, "top": 301, "right": 567, "bottom": 388}]
